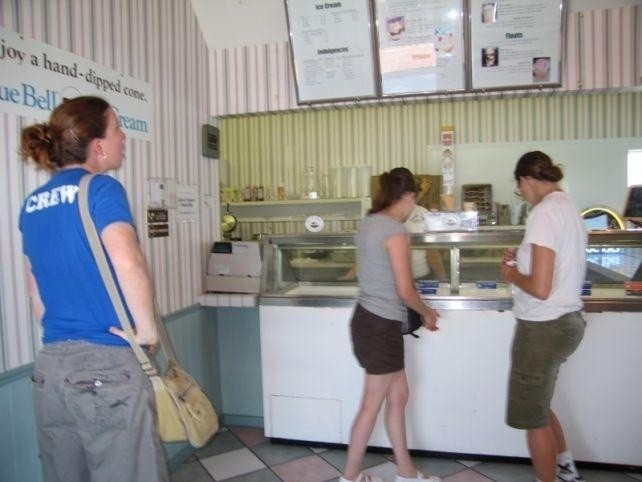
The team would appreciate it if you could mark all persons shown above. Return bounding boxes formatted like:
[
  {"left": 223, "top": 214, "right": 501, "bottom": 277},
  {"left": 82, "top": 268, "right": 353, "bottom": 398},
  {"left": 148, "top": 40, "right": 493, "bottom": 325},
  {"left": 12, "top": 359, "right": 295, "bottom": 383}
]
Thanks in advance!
[
  {"left": 339, "top": 168, "right": 441, "bottom": 482},
  {"left": 336, "top": 173, "right": 449, "bottom": 282},
  {"left": 500, "top": 151, "right": 586, "bottom": 482},
  {"left": 19, "top": 97, "right": 169, "bottom": 482}
]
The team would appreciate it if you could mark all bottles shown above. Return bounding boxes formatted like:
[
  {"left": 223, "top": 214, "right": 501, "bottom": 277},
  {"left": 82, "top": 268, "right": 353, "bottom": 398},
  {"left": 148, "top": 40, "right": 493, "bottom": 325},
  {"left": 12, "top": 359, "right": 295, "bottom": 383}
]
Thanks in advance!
[{"left": 239, "top": 182, "right": 289, "bottom": 202}]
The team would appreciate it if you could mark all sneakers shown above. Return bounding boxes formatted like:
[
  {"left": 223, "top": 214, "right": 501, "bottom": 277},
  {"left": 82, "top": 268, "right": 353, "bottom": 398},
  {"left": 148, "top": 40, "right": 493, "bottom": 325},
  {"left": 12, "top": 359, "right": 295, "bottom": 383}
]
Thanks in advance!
[
  {"left": 556, "top": 462, "right": 587, "bottom": 482},
  {"left": 338, "top": 470, "right": 385, "bottom": 482},
  {"left": 394, "top": 470, "right": 443, "bottom": 482}
]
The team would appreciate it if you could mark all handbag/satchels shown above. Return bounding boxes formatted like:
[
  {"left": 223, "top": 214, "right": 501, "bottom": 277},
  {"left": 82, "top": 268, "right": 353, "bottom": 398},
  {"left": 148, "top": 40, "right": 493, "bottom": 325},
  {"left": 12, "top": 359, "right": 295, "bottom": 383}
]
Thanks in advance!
[
  {"left": 402, "top": 307, "right": 422, "bottom": 336},
  {"left": 152, "top": 363, "right": 220, "bottom": 448}
]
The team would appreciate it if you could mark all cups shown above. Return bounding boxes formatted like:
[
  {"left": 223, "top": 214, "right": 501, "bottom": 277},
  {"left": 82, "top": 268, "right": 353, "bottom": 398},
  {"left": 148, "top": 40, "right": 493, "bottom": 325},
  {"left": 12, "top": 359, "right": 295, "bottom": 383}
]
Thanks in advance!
[
  {"left": 297, "top": 167, "right": 322, "bottom": 199},
  {"left": 328, "top": 165, "right": 373, "bottom": 198}
]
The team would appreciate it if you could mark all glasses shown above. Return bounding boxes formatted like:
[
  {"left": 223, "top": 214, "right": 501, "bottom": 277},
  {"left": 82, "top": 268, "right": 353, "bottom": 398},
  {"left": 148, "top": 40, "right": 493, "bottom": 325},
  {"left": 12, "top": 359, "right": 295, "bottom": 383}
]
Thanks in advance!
[{"left": 513, "top": 186, "right": 524, "bottom": 200}]
